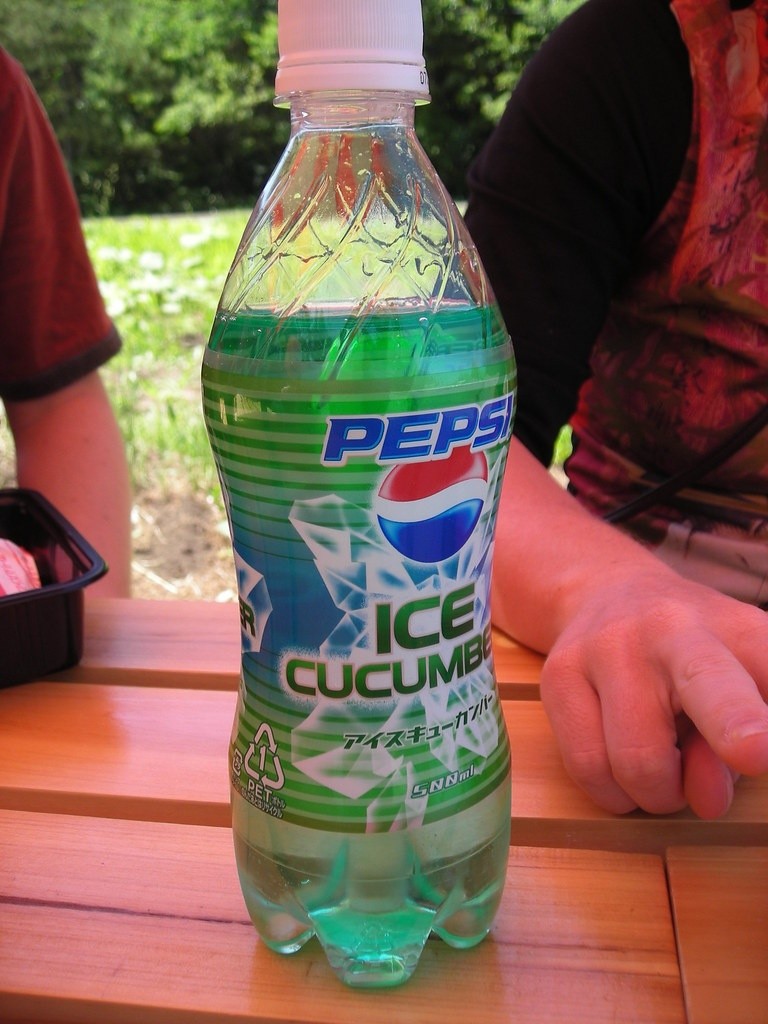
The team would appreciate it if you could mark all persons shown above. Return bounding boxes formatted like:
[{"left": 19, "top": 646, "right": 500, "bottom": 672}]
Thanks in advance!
[
  {"left": 403, "top": 1, "right": 768, "bottom": 823},
  {"left": 0, "top": 43, "right": 132, "bottom": 599}
]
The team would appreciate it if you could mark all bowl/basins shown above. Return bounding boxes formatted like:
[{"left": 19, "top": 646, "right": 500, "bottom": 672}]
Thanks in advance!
[{"left": 0, "top": 487, "right": 108, "bottom": 687}]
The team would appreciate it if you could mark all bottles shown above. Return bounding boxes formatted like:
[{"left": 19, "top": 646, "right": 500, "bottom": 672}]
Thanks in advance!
[{"left": 201, "top": 1, "right": 518, "bottom": 989}]
